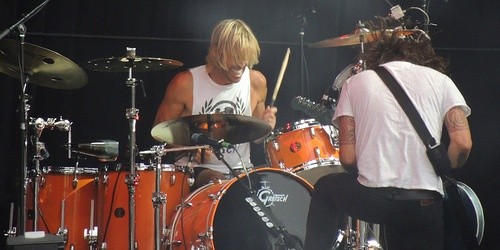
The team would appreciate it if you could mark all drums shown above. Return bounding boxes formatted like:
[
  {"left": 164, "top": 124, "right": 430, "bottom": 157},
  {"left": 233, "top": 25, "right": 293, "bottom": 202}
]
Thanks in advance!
[
  {"left": 26, "top": 167, "right": 107, "bottom": 250},
  {"left": 232, "top": 168, "right": 254, "bottom": 179},
  {"left": 97, "top": 163, "right": 192, "bottom": 249},
  {"left": 164, "top": 168, "right": 314, "bottom": 250},
  {"left": 266, "top": 119, "right": 350, "bottom": 187}
]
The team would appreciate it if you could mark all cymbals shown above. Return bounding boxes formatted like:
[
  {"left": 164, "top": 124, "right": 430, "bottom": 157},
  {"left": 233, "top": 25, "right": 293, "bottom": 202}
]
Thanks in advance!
[
  {"left": 151, "top": 114, "right": 272, "bottom": 146},
  {"left": 306, "top": 28, "right": 418, "bottom": 49},
  {"left": 0, "top": 39, "right": 89, "bottom": 90},
  {"left": 86, "top": 58, "right": 185, "bottom": 74}
]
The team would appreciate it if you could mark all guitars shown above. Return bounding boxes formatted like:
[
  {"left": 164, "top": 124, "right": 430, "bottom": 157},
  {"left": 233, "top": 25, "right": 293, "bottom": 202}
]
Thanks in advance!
[{"left": 290, "top": 95, "right": 484, "bottom": 245}]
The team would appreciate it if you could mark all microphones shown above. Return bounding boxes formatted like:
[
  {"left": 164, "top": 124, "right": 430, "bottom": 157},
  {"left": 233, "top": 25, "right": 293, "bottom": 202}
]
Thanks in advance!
[{"left": 192, "top": 132, "right": 237, "bottom": 149}]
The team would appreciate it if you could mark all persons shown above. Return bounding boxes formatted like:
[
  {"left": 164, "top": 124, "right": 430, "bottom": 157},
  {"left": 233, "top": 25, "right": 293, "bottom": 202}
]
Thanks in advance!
[
  {"left": 154, "top": 19, "right": 278, "bottom": 190},
  {"left": 305, "top": 38, "right": 472, "bottom": 250}
]
